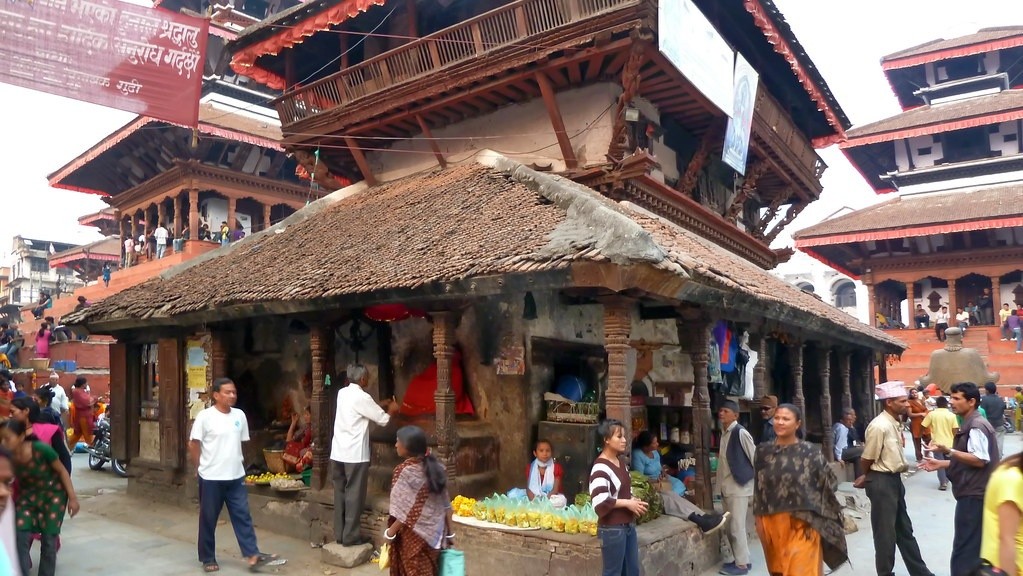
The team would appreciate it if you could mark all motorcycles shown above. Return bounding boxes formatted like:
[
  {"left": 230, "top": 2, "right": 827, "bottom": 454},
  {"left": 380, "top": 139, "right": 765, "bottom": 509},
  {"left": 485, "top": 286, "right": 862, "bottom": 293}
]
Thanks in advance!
[{"left": 83, "top": 416, "right": 128, "bottom": 478}]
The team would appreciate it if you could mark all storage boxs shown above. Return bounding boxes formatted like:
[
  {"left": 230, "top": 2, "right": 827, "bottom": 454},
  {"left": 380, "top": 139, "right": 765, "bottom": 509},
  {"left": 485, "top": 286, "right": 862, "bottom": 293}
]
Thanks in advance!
[{"left": 54, "top": 361, "right": 77, "bottom": 372}]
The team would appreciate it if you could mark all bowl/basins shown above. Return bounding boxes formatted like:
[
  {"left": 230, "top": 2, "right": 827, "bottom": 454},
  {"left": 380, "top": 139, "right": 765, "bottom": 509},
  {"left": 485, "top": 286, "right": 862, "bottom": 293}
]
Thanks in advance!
[{"left": 301, "top": 469, "right": 312, "bottom": 486}]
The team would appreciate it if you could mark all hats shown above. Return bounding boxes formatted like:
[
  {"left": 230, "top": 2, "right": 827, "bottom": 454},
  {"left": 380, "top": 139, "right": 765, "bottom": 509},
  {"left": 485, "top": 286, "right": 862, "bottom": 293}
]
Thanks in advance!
[
  {"left": 761, "top": 395, "right": 777, "bottom": 407},
  {"left": 875, "top": 381, "right": 908, "bottom": 400},
  {"left": 721, "top": 399, "right": 739, "bottom": 413}
]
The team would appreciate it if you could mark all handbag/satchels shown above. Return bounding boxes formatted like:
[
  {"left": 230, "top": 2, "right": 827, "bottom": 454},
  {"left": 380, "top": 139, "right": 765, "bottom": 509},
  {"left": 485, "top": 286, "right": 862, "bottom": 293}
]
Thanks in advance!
[{"left": 441, "top": 550, "right": 464, "bottom": 576}]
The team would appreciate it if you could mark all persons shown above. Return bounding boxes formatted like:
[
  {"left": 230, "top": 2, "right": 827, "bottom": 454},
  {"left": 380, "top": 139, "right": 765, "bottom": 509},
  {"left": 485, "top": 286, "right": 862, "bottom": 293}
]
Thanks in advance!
[
  {"left": 979, "top": 452, "right": 1023, "bottom": 576},
  {"left": 632, "top": 429, "right": 730, "bottom": 535},
  {"left": 915, "top": 382, "right": 999, "bottom": 576},
  {"left": 832, "top": 380, "right": 1023, "bottom": 489},
  {"left": 875, "top": 286, "right": 1023, "bottom": 354},
  {"left": 0, "top": 290, "right": 113, "bottom": 576},
  {"left": 853, "top": 381, "right": 935, "bottom": 576},
  {"left": 588, "top": 418, "right": 649, "bottom": 576},
  {"left": 714, "top": 399, "right": 757, "bottom": 576},
  {"left": 121, "top": 205, "right": 246, "bottom": 270},
  {"left": 281, "top": 403, "right": 314, "bottom": 476},
  {"left": 329, "top": 363, "right": 399, "bottom": 547},
  {"left": 749, "top": 394, "right": 777, "bottom": 446},
  {"left": 918, "top": 326, "right": 1000, "bottom": 394},
  {"left": 382, "top": 425, "right": 457, "bottom": 576},
  {"left": 101, "top": 261, "right": 113, "bottom": 288},
  {"left": 753, "top": 403, "right": 851, "bottom": 576},
  {"left": 188, "top": 377, "right": 279, "bottom": 572},
  {"left": 526, "top": 439, "right": 563, "bottom": 501}
]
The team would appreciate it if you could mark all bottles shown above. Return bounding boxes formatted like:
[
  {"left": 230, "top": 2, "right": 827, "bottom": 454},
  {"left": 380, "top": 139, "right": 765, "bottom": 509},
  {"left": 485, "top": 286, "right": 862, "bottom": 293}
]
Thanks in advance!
[
  {"left": 709, "top": 452, "right": 718, "bottom": 473},
  {"left": 581, "top": 390, "right": 596, "bottom": 402}
]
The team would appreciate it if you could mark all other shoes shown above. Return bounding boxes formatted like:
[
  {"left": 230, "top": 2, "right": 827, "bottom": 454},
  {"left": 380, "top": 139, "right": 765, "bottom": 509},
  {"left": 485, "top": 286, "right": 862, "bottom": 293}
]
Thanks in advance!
[
  {"left": 938, "top": 484, "right": 947, "bottom": 490},
  {"left": 343, "top": 536, "right": 371, "bottom": 546},
  {"left": 723, "top": 560, "right": 752, "bottom": 570},
  {"left": 703, "top": 517, "right": 726, "bottom": 535},
  {"left": 720, "top": 512, "right": 732, "bottom": 521},
  {"left": 720, "top": 566, "right": 748, "bottom": 576}
]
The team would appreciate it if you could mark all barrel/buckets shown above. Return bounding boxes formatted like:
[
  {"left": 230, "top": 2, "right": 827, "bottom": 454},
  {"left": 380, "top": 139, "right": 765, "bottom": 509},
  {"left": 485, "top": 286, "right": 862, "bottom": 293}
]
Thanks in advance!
[{"left": 558, "top": 373, "right": 589, "bottom": 401}]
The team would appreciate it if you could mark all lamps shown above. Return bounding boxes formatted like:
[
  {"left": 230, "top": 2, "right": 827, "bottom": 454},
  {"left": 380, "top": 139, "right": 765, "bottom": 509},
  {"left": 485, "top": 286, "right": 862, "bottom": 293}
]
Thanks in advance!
[
  {"left": 645, "top": 114, "right": 665, "bottom": 143},
  {"left": 624, "top": 106, "right": 640, "bottom": 122}
]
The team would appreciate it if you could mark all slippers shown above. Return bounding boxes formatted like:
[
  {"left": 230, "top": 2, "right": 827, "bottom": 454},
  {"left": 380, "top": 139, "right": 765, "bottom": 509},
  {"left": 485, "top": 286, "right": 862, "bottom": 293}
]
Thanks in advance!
[
  {"left": 252, "top": 553, "right": 279, "bottom": 570},
  {"left": 203, "top": 559, "right": 219, "bottom": 573}
]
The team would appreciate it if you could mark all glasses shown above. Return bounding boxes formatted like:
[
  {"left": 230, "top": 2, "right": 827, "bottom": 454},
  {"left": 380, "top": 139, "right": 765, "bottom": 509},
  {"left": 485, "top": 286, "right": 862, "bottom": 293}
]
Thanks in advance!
[{"left": 760, "top": 406, "right": 771, "bottom": 411}]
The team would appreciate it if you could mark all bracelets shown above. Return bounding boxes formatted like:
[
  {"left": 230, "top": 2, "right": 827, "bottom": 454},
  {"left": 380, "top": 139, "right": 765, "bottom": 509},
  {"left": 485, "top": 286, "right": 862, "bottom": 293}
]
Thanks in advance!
[
  {"left": 446, "top": 533, "right": 455, "bottom": 538},
  {"left": 384, "top": 529, "right": 395, "bottom": 539},
  {"left": 945, "top": 449, "right": 955, "bottom": 457}
]
